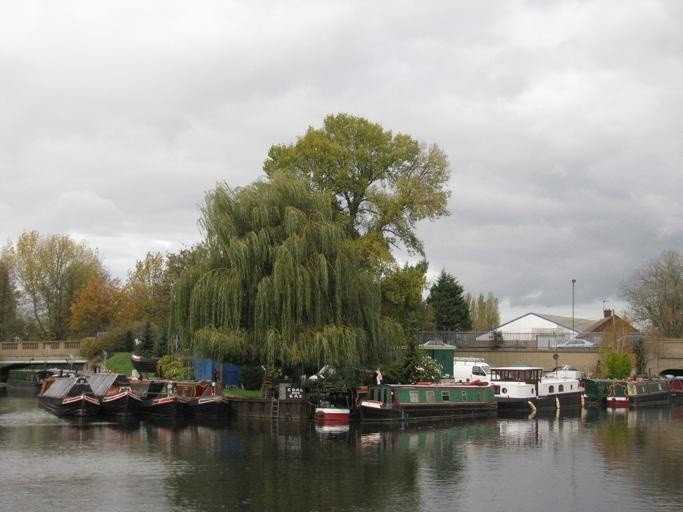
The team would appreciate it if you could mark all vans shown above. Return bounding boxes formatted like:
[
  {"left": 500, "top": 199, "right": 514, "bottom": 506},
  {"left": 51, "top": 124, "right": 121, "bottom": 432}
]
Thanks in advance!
[{"left": 453, "top": 361, "right": 500, "bottom": 382}]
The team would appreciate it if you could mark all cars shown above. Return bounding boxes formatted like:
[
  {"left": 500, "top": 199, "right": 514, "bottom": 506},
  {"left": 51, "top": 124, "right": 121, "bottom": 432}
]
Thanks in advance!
[
  {"left": 553, "top": 339, "right": 598, "bottom": 350},
  {"left": 419, "top": 340, "right": 456, "bottom": 349}
]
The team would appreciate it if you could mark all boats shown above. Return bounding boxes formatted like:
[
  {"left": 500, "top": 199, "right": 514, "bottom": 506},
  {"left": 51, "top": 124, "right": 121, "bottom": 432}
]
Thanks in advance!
[
  {"left": 37, "top": 370, "right": 230, "bottom": 418},
  {"left": 315, "top": 408, "right": 350, "bottom": 426}
]
